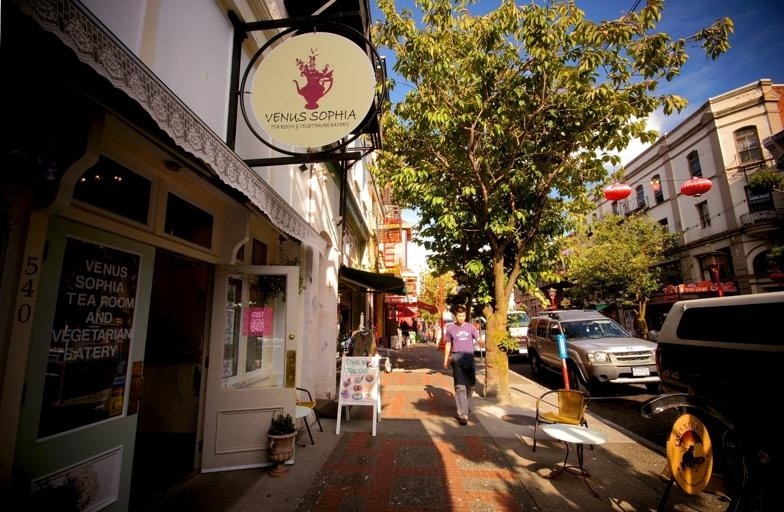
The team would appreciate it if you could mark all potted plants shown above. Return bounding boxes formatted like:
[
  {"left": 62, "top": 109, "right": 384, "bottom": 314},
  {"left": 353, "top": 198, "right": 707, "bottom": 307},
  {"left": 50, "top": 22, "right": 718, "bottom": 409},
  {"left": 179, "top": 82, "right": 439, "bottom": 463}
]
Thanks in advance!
[{"left": 261, "top": 410, "right": 296, "bottom": 480}]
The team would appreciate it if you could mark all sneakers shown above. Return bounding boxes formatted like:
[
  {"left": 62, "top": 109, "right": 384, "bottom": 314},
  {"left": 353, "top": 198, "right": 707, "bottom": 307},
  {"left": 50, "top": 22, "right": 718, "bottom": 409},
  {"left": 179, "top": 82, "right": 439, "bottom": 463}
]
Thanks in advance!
[{"left": 458, "top": 418, "right": 467, "bottom": 426}]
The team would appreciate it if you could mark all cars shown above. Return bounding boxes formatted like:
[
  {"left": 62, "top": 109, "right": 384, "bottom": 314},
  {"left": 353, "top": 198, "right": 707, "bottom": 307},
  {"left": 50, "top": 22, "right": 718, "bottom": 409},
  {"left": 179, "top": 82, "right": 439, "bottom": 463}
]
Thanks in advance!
[{"left": 472, "top": 311, "right": 533, "bottom": 363}]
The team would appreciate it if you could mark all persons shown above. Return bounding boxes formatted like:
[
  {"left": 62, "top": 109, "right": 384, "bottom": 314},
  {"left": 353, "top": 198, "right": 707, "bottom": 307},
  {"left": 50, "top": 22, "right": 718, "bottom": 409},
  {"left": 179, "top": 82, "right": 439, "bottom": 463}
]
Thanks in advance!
[
  {"left": 401, "top": 315, "right": 440, "bottom": 343},
  {"left": 442, "top": 305, "right": 487, "bottom": 426}
]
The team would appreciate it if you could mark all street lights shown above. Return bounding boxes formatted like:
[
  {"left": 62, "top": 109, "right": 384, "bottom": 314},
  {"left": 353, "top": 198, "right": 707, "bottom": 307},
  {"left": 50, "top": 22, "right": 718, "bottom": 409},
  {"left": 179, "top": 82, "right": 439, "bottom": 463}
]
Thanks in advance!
[{"left": 698, "top": 249, "right": 728, "bottom": 297}]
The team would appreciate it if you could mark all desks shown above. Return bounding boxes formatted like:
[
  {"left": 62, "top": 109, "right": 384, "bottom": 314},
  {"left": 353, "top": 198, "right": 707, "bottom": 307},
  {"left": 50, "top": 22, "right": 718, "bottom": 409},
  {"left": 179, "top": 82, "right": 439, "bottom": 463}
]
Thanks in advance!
[{"left": 540, "top": 423, "right": 606, "bottom": 499}]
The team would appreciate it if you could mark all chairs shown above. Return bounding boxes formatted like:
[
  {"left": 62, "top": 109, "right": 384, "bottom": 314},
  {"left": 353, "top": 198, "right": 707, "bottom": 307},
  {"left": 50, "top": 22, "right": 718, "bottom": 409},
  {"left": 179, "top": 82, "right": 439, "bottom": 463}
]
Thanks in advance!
[
  {"left": 292, "top": 388, "right": 323, "bottom": 447},
  {"left": 531, "top": 389, "right": 594, "bottom": 454}
]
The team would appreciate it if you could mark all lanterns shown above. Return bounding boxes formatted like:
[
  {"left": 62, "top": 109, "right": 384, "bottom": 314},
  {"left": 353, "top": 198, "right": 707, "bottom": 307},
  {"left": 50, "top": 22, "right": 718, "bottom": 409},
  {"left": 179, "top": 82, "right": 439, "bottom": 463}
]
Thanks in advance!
[
  {"left": 679, "top": 174, "right": 714, "bottom": 199},
  {"left": 769, "top": 271, "right": 784, "bottom": 283},
  {"left": 603, "top": 181, "right": 633, "bottom": 203}
]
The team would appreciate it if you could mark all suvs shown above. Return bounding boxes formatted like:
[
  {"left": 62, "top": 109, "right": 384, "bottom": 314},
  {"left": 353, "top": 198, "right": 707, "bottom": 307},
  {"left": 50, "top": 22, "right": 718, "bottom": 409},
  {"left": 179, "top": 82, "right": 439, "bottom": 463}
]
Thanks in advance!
[
  {"left": 525, "top": 308, "right": 662, "bottom": 399},
  {"left": 656, "top": 291, "right": 784, "bottom": 494}
]
